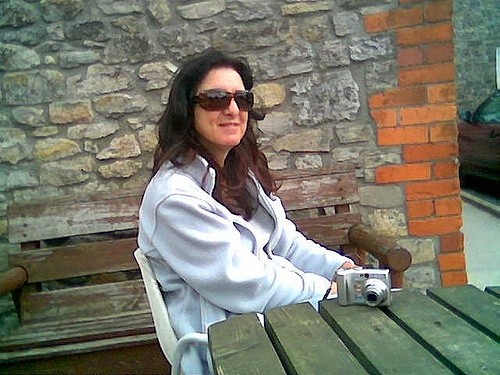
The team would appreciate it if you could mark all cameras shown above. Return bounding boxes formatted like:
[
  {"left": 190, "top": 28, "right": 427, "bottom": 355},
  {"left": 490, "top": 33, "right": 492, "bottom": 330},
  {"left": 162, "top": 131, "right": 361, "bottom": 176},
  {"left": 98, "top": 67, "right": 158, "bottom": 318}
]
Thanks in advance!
[{"left": 336, "top": 266, "right": 392, "bottom": 307}]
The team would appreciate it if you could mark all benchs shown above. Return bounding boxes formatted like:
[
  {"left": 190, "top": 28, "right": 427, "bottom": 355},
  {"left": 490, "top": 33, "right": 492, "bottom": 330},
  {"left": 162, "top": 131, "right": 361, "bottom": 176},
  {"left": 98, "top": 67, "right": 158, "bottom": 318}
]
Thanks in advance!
[{"left": 0, "top": 163, "right": 411, "bottom": 375}]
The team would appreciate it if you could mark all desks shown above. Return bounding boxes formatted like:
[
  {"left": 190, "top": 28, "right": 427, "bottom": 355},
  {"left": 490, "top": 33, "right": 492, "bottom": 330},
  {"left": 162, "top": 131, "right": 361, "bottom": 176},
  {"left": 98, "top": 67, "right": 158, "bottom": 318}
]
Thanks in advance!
[{"left": 209, "top": 283, "right": 499, "bottom": 375}]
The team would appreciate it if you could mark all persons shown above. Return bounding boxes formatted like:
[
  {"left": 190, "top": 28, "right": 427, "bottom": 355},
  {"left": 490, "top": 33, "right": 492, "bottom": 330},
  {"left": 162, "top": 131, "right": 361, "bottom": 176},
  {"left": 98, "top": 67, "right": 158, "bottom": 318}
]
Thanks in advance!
[{"left": 137, "top": 51, "right": 362, "bottom": 375}]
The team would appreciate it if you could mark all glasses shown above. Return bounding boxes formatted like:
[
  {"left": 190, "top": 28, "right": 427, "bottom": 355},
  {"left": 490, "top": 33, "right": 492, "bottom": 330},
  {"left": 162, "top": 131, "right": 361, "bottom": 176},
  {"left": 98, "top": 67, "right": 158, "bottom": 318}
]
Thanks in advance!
[{"left": 193, "top": 88, "right": 254, "bottom": 111}]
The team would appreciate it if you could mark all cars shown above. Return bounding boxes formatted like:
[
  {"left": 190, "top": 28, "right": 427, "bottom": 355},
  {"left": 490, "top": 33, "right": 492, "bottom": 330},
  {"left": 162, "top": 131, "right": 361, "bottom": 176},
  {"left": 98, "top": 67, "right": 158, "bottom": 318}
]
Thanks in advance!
[{"left": 459, "top": 89, "right": 500, "bottom": 197}]
